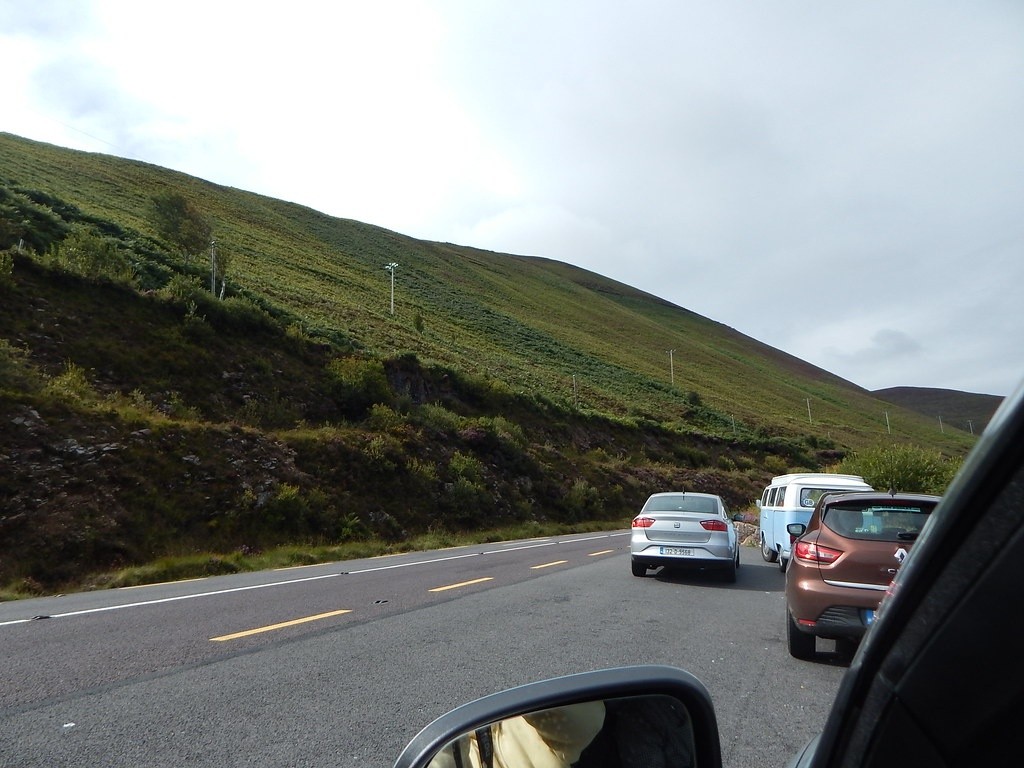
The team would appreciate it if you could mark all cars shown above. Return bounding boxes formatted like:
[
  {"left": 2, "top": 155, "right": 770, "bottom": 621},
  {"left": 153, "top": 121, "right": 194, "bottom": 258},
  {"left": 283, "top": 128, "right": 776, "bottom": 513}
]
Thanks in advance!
[{"left": 631, "top": 492, "right": 743, "bottom": 580}]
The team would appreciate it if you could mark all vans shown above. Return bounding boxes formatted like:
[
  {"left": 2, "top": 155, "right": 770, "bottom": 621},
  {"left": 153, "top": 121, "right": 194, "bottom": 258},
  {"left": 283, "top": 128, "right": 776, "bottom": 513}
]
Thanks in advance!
[{"left": 756, "top": 473, "right": 876, "bottom": 571}]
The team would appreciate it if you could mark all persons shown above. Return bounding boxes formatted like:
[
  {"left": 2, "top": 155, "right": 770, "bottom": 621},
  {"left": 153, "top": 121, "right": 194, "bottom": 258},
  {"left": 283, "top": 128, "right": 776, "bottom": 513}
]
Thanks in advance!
[{"left": 465, "top": 699, "right": 606, "bottom": 767}]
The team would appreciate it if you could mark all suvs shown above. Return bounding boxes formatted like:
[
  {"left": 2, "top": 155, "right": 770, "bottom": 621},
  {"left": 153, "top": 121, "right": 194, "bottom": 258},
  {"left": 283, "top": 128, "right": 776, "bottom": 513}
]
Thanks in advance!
[{"left": 784, "top": 488, "right": 949, "bottom": 659}]
